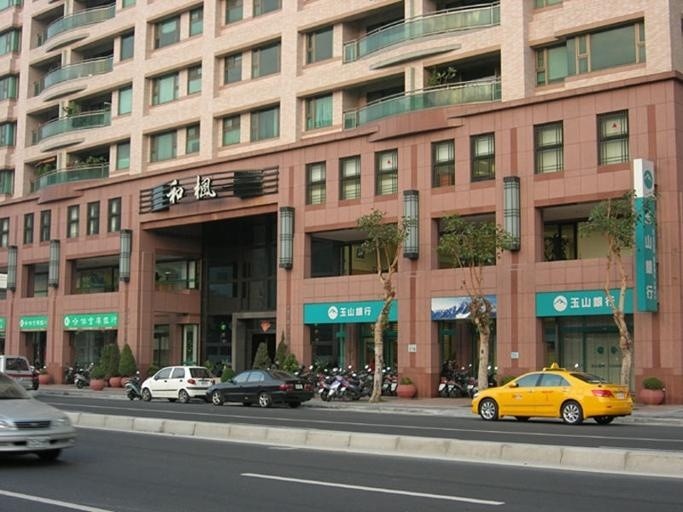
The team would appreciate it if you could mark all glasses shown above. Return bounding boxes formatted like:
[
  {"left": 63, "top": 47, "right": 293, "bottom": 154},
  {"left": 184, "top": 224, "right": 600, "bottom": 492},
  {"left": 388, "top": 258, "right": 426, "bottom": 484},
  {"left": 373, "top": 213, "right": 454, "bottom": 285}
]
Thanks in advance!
[
  {"left": 277, "top": 206, "right": 294, "bottom": 269},
  {"left": 47, "top": 239, "right": 60, "bottom": 289},
  {"left": 503, "top": 175, "right": 519, "bottom": 251},
  {"left": 6, "top": 244, "right": 17, "bottom": 292},
  {"left": 401, "top": 189, "right": 420, "bottom": 261},
  {"left": 118, "top": 228, "right": 132, "bottom": 283}
]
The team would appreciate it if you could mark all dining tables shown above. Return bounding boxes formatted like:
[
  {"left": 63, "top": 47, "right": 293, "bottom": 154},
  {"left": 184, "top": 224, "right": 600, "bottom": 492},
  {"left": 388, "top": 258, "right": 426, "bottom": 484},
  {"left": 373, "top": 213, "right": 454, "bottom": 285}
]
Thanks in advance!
[
  {"left": 88, "top": 341, "right": 137, "bottom": 391},
  {"left": 38, "top": 367, "right": 50, "bottom": 385},
  {"left": 395, "top": 376, "right": 418, "bottom": 399},
  {"left": 638, "top": 377, "right": 666, "bottom": 406}
]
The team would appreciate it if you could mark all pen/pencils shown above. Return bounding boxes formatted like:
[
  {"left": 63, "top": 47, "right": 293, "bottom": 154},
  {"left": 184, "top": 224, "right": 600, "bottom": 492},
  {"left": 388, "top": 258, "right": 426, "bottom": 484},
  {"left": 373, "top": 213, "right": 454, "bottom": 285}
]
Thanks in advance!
[{"left": 0, "top": 354, "right": 33, "bottom": 392}]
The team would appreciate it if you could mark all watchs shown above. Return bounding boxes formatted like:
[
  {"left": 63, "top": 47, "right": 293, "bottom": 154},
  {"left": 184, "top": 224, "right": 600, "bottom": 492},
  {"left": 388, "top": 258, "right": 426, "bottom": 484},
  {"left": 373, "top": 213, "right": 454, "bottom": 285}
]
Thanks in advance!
[
  {"left": 437, "top": 358, "right": 499, "bottom": 399},
  {"left": 274, "top": 361, "right": 397, "bottom": 401},
  {"left": 123, "top": 370, "right": 144, "bottom": 400},
  {"left": 65, "top": 360, "right": 94, "bottom": 389}
]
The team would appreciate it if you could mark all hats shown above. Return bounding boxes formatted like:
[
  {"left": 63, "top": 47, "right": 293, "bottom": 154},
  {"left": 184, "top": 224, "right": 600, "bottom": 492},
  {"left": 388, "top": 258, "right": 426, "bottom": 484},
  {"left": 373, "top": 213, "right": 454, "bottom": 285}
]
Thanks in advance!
[
  {"left": 202, "top": 367, "right": 315, "bottom": 409},
  {"left": 30, "top": 364, "right": 41, "bottom": 390},
  {"left": 471, "top": 361, "right": 639, "bottom": 425},
  {"left": 0, "top": 371, "right": 78, "bottom": 464},
  {"left": 139, "top": 364, "right": 222, "bottom": 402}
]
